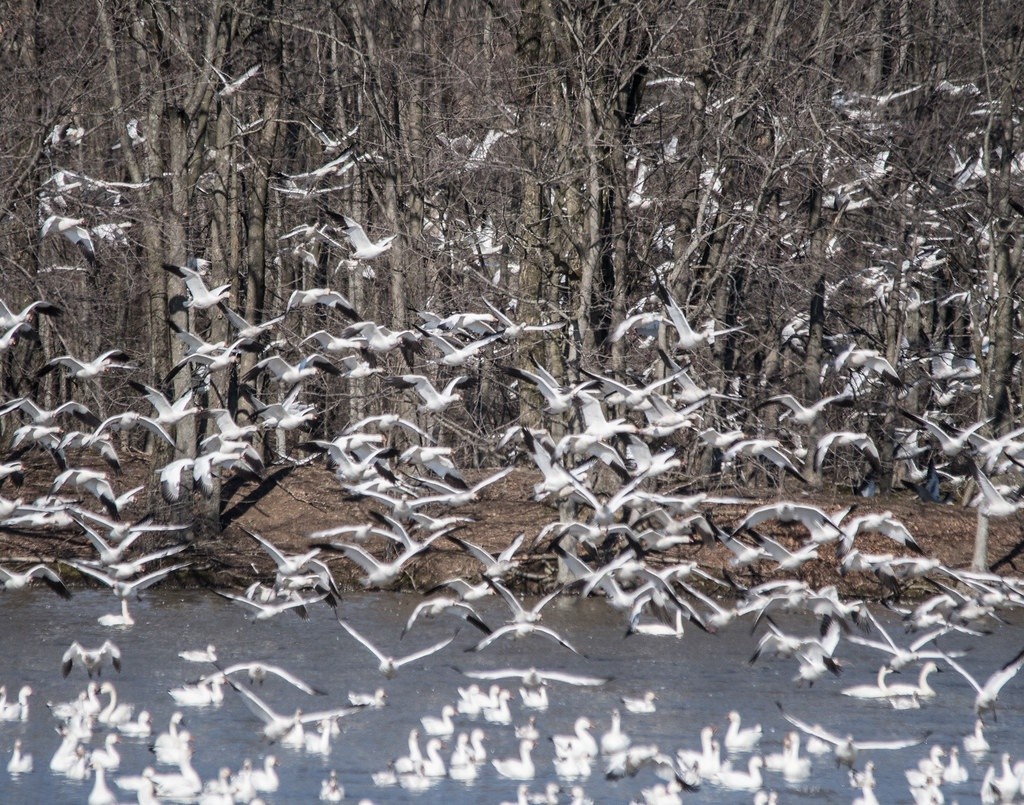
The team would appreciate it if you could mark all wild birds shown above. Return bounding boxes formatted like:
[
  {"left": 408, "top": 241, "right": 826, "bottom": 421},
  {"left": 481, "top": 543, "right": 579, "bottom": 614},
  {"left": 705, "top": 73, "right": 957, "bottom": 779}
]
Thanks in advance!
[{"left": 0, "top": 64, "right": 1022, "bottom": 804}]
